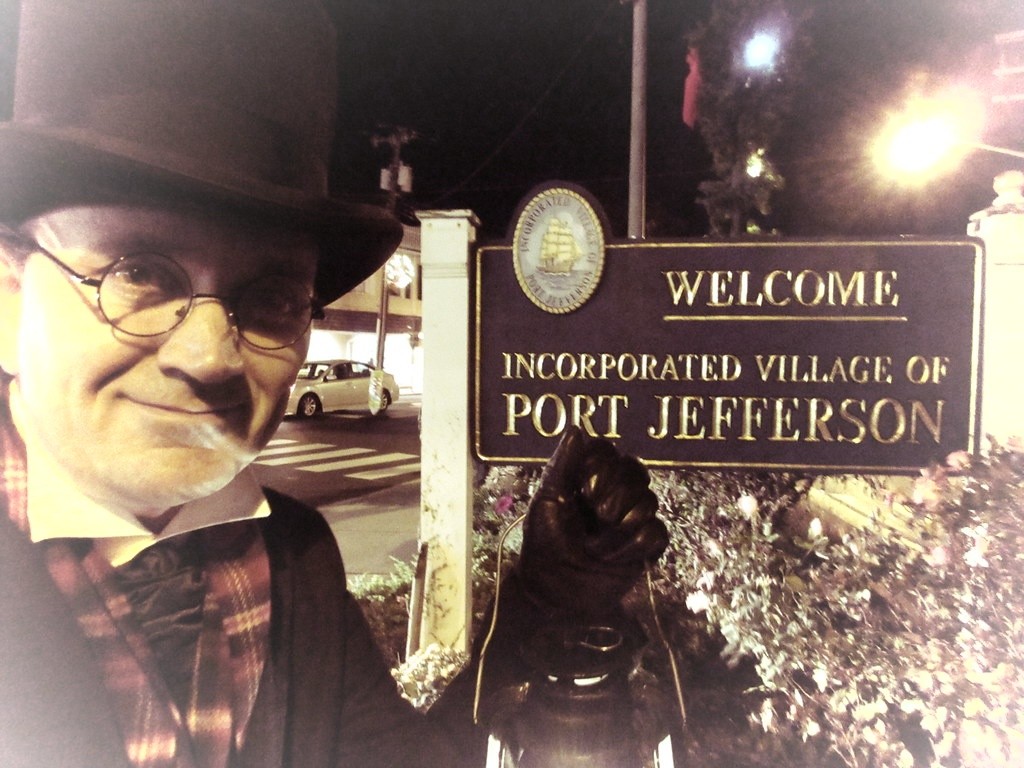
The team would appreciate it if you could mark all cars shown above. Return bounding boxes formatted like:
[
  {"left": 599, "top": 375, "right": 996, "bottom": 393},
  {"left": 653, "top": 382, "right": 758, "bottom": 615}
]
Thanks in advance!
[{"left": 282, "top": 359, "right": 399, "bottom": 420}]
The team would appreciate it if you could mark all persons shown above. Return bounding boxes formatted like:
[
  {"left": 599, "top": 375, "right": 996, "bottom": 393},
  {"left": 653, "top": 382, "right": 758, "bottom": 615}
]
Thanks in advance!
[{"left": 0, "top": 1, "right": 668, "bottom": 768}]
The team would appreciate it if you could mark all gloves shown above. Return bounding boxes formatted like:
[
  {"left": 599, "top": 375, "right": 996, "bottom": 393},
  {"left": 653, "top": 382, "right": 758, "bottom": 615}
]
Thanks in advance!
[{"left": 516, "top": 426, "right": 669, "bottom": 620}]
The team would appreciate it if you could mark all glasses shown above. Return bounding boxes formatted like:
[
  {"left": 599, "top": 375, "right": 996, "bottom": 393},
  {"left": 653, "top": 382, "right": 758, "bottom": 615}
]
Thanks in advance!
[{"left": 10, "top": 225, "right": 325, "bottom": 350}]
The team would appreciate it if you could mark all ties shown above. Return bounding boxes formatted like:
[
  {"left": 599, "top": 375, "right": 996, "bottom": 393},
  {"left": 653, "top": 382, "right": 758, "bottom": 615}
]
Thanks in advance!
[{"left": 123, "top": 545, "right": 205, "bottom": 714}]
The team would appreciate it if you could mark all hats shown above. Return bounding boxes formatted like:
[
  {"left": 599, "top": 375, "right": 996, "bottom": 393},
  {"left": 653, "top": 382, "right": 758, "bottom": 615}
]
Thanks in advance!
[{"left": 0, "top": 0, "right": 404, "bottom": 311}]
